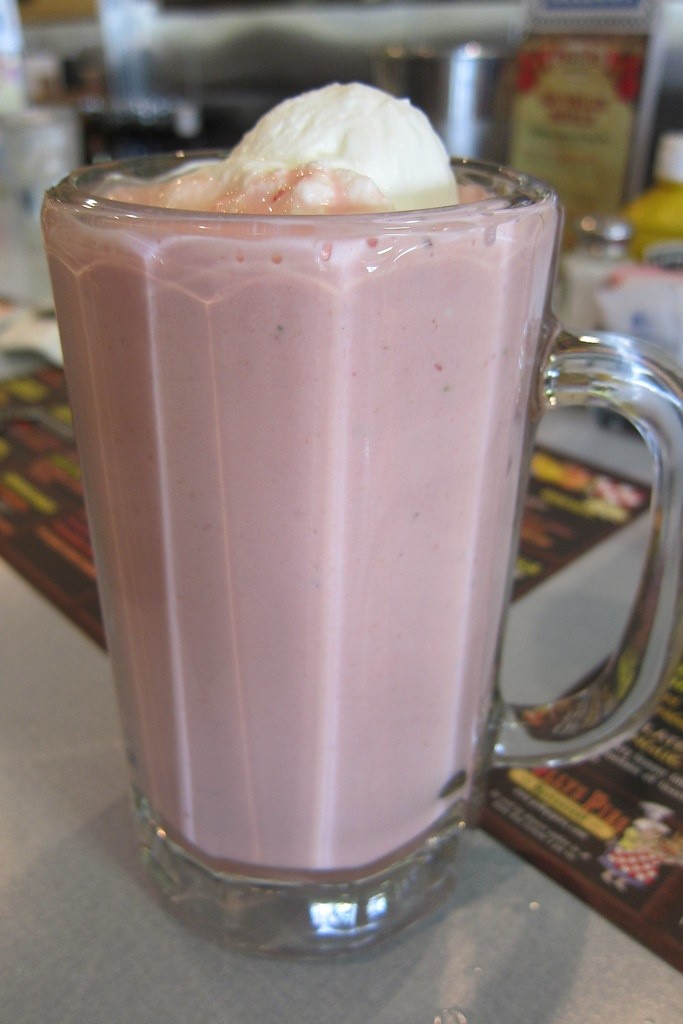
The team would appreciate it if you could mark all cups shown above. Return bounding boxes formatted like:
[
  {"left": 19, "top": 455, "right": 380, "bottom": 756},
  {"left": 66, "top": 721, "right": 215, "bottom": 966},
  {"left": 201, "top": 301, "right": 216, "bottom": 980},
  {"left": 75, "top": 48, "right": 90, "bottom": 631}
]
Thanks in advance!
[{"left": 38, "top": 150, "right": 681, "bottom": 965}]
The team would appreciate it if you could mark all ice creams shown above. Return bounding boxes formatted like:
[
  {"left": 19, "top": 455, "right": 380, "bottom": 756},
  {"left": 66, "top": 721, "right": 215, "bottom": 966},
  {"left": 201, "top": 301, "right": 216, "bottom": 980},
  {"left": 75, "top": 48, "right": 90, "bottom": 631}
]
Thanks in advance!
[{"left": 96, "top": 82, "right": 460, "bottom": 222}]
